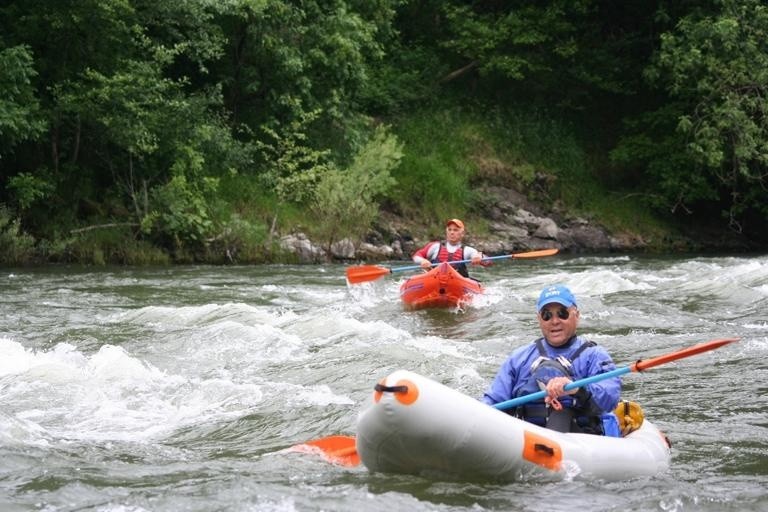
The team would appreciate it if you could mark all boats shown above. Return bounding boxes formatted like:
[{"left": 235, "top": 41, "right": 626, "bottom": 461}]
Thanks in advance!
[
  {"left": 354, "top": 369, "right": 672, "bottom": 481},
  {"left": 401, "top": 262, "right": 485, "bottom": 310}
]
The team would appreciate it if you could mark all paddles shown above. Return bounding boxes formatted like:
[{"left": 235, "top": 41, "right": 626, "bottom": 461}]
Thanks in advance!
[
  {"left": 346, "top": 248, "right": 559, "bottom": 282},
  {"left": 295, "top": 337, "right": 741, "bottom": 471}
]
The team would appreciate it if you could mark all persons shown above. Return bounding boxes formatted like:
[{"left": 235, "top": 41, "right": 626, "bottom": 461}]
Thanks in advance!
[
  {"left": 482, "top": 285, "right": 623, "bottom": 436},
  {"left": 411, "top": 218, "right": 493, "bottom": 277}
]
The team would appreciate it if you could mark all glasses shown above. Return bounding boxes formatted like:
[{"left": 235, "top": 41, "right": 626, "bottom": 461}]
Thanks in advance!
[{"left": 541, "top": 308, "right": 570, "bottom": 321}]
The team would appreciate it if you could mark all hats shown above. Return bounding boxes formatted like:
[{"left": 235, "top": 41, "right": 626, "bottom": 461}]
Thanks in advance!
[
  {"left": 447, "top": 219, "right": 464, "bottom": 228},
  {"left": 537, "top": 285, "right": 577, "bottom": 312}
]
